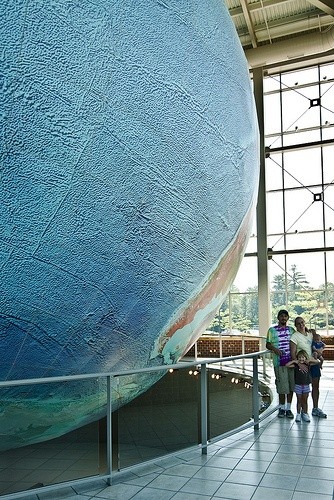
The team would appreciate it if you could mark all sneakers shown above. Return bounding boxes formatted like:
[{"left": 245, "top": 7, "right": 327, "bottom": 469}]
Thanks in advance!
[
  {"left": 295, "top": 410, "right": 311, "bottom": 422},
  {"left": 312, "top": 408, "right": 328, "bottom": 418},
  {"left": 278, "top": 409, "right": 294, "bottom": 418}
]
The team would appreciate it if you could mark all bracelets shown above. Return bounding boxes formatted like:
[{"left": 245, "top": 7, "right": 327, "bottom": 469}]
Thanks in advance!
[{"left": 297, "top": 362, "right": 300, "bottom": 365}]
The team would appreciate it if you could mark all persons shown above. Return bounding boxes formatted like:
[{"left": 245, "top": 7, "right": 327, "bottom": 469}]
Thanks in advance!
[
  {"left": 312, "top": 334, "right": 325, "bottom": 370},
  {"left": 266, "top": 310, "right": 296, "bottom": 418},
  {"left": 286, "top": 350, "right": 318, "bottom": 422},
  {"left": 290, "top": 317, "right": 327, "bottom": 418},
  {"left": 219, "top": 325, "right": 225, "bottom": 333}
]
